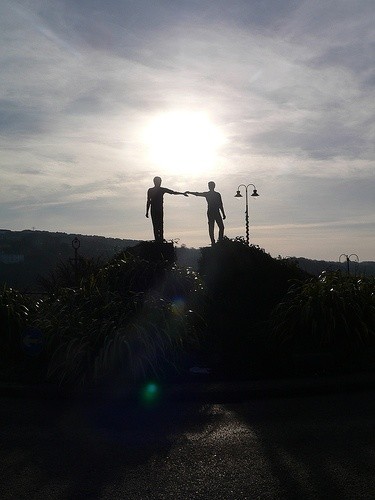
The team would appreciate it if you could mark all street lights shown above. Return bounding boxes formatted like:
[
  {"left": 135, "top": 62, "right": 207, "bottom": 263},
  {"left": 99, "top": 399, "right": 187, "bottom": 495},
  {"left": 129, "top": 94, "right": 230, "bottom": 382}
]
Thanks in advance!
[
  {"left": 338, "top": 252, "right": 359, "bottom": 276},
  {"left": 234, "top": 183, "right": 260, "bottom": 246},
  {"left": 72, "top": 237, "right": 80, "bottom": 268}
]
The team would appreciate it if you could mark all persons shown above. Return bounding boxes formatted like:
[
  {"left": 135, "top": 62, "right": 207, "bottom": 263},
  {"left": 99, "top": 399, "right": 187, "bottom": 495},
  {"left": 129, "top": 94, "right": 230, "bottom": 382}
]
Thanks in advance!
[
  {"left": 185, "top": 181, "right": 226, "bottom": 246},
  {"left": 145, "top": 177, "right": 188, "bottom": 240}
]
